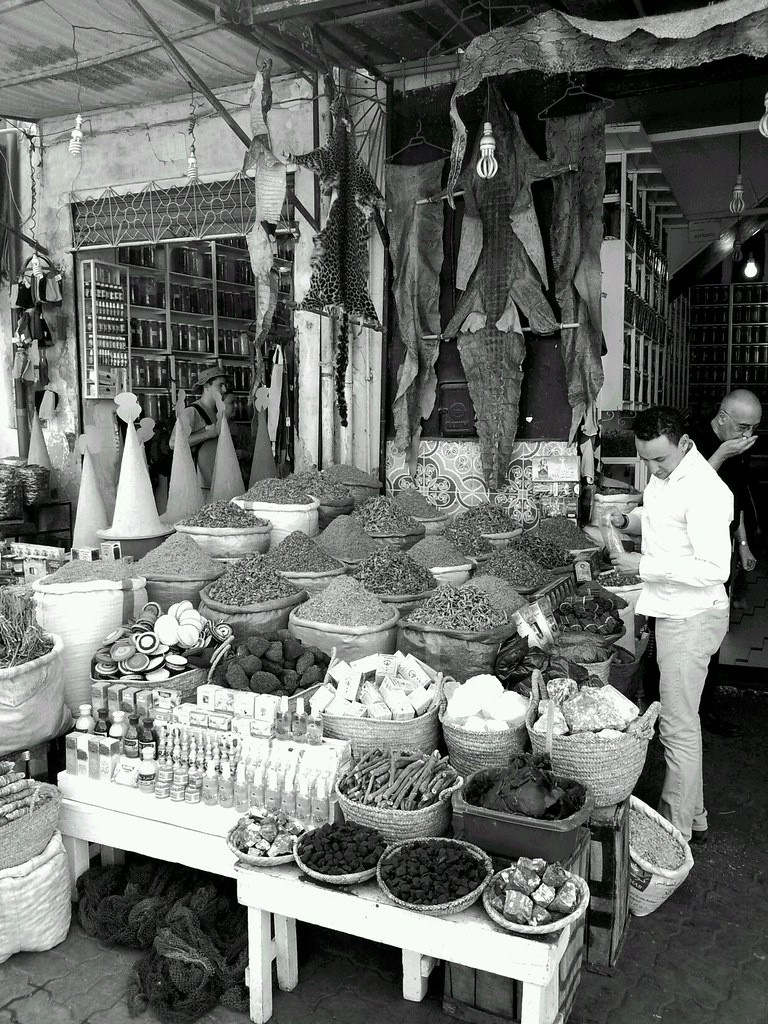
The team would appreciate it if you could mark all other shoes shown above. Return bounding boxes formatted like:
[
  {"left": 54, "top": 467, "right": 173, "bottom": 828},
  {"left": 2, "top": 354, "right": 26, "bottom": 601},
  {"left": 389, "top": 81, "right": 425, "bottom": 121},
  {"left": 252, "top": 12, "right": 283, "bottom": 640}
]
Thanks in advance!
[{"left": 692, "top": 816, "right": 708, "bottom": 832}]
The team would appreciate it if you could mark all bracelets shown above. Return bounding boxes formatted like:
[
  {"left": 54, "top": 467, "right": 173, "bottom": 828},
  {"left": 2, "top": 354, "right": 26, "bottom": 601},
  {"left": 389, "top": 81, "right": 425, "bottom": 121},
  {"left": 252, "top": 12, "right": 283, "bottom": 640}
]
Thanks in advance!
[
  {"left": 247, "top": 451, "right": 252, "bottom": 461},
  {"left": 610, "top": 513, "right": 629, "bottom": 529}
]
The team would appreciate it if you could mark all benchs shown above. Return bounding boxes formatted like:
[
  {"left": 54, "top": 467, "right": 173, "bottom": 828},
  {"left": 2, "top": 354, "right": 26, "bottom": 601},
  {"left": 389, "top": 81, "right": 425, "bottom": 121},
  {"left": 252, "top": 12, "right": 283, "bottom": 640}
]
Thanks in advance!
[
  {"left": 81, "top": 259, "right": 132, "bottom": 399},
  {"left": 234, "top": 860, "right": 569, "bottom": 1024},
  {"left": 56, "top": 799, "right": 316, "bottom": 902}
]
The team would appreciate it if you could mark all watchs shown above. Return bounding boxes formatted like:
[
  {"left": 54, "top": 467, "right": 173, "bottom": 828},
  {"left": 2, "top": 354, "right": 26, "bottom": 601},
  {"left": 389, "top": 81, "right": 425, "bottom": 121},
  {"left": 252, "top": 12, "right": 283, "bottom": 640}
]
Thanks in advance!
[{"left": 739, "top": 540, "right": 747, "bottom": 545}]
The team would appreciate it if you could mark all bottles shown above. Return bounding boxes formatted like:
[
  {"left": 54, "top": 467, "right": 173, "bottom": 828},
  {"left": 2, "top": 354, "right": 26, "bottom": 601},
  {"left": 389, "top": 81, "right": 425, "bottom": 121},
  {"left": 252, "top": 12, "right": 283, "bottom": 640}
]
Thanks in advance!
[
  {"left": 275, "top": 696, "right": 291, "bottom": 741},
  {"left": 75, "top": 703, "right": 96, "bottom": 735},
  {"left": 136, "top": 746, "right": 330, "bottom": 830},
  {"left": 120, "top": 239, "right": 293, "bottom": 421},
  {"left": 108, "top": 711, "right": 128, "bottom": 755},
  {"left": 124, "top": 713, "right": 141, "bottom": 758},
  {"left": 291, "top": 697, "right": 308, "bottom": 744},
  {"left": 138, "top": 717, "right": 158, "bottom": 761},
  {"left": 93, "top": 708, "right": 112, "bottom": 737},
  {"left": 306, "top": 701, "right": 324, "bottom": 745}
]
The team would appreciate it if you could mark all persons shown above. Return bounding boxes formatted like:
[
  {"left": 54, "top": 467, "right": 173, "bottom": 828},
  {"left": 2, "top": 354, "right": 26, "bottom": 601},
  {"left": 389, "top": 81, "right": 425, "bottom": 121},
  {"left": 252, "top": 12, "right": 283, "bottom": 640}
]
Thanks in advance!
[
  {"left": 694, "top": 389, "right": 761, "bottom": 571},
  {"left": 169, "top": 367, "right": 253, "bottom": 506},
  {"left": 597, "top": 405, "right": 734, "bottom": 847}
]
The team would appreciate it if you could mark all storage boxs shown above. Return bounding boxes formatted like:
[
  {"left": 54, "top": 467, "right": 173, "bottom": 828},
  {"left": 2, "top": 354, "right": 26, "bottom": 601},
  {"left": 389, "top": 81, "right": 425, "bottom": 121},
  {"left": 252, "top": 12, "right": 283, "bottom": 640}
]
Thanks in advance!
[{"left": 451, "top": 767, "right": 596, "bottom": 865}]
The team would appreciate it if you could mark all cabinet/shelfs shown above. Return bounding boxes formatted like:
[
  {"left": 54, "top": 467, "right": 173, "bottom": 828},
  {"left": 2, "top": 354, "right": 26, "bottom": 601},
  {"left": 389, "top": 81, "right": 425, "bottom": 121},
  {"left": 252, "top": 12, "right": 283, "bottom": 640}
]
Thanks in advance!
[
  {"left": 116, "top": 240, "right": 292, "bottom": 424},
  {"left": 595, "top": 121, "right": 686, "bottom": 473},
  {"left": 686, "top": 282, "right": 768, "bottom": 411},
  {"left": 0, "top": 497, "right": 73, "bottom": 553}
]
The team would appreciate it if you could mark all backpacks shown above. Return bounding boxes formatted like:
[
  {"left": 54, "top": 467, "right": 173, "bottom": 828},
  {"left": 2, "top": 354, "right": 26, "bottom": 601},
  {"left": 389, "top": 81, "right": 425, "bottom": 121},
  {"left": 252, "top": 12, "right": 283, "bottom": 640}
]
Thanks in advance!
[{"left": 157, "top": 403, "right": 214, "bottom": 476}]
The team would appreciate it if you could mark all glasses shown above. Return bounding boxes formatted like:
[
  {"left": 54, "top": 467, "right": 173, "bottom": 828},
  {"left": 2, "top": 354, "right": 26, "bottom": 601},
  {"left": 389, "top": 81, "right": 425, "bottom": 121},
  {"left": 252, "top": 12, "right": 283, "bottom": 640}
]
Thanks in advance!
[{"left": 721, "top": 409, "right": 762, "bottom": 432}]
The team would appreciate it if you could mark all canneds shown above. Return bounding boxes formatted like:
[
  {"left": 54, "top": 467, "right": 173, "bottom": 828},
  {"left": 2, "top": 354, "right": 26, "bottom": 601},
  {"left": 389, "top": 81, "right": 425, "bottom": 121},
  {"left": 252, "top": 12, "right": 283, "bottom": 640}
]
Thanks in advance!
[
  {"left": 119, "top": 238, "right": 290, "bottom": 425},
  {"left": 689, "top": 284, "right": 768, "bottom": 402}
]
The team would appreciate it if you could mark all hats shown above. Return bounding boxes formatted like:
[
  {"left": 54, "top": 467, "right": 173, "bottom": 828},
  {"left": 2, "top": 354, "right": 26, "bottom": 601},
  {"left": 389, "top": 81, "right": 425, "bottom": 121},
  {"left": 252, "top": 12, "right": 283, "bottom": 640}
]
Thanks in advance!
[{"left": 192, "top": 366, "right": 233, "bottom": 390}]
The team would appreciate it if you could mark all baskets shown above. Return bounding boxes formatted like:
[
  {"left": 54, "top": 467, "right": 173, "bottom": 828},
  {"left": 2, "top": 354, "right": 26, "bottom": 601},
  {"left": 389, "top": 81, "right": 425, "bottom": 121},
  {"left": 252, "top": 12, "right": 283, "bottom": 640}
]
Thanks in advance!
[
  {"left": 90, "top": 635, "right": 234, "bottom": 705},
  {"left": 439, "top": 675, "right": 533, "bottom": 780},
  {"left": 319, "top": 658, "right": 443, "bottom": 758},
  {"left": 526, "top": 669, "right": 662, "bottom": 807},
  {"left": 0, "top": 782, "right": 63, "bottom": 870},
  {"left": 208, "top": 644, "right": 325, "bottom": 713},
  {"left": 335, "top": 751, "right": 464, "bottom": 842}
]
